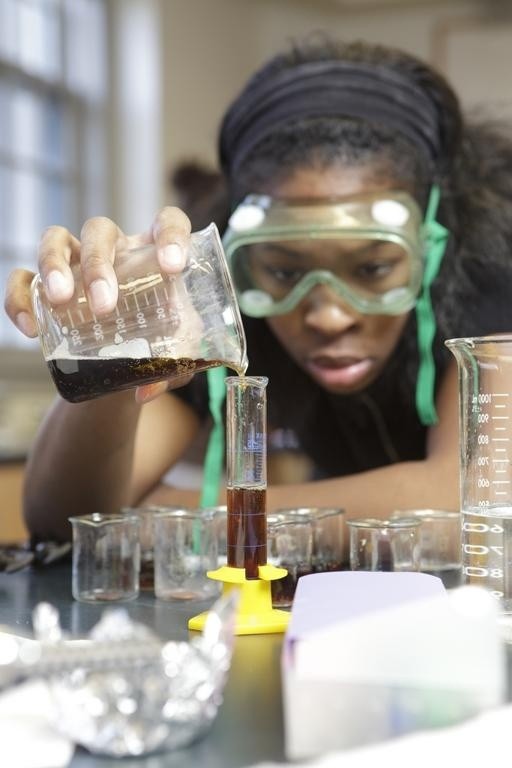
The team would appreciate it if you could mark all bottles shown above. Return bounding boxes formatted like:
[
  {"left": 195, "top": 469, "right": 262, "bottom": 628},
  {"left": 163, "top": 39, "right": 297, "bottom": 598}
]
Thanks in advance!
[
  {"left": 445, "top": 333, "right": 512, "bottom": 706},
  {"left": 224, "top": 375, "right": 269, "bottom": 578}
]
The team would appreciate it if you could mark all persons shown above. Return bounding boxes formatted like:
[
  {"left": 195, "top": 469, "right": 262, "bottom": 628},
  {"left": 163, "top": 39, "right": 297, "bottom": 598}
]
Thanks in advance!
[
  {"left": 4, "top": 32, "right": 511, "bottom": 560},
  {"left": 169, "top": 156, "right": 230, "bottom": 234}
]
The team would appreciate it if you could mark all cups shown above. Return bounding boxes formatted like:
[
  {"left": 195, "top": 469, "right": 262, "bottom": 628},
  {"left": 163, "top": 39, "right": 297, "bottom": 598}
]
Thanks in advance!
[
  {"left": 280, "top": 507, "right": 345, "bottom": 575},
  {"left": 151, "top": 508, "right": 218, "bottom": 601},
  {"left": 393, "top": 509, "right": 463, "bottom": 587},
  {"left": 212, "top": 504, "right": 236, "bottom": 590},
  {"left": 345, "top": 517, "right": 425, "bottom": 573},
  {"left": 127, "top": 505, "right": 177, "bottom": 591},
  {"left": 31, "top": 221, "right": 249, "bottom": 403},
  {"left": 66, "top": 509, "right": 142, "bottom": 606},
  {"left": 261, "top": 513, "right": 315, "bottom": 609}
]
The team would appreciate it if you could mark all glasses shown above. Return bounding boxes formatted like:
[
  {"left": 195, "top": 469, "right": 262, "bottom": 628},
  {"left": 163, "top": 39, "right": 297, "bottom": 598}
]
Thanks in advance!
[{"left": 224, "top": 191, "right": 426, "bottom": 319}]
